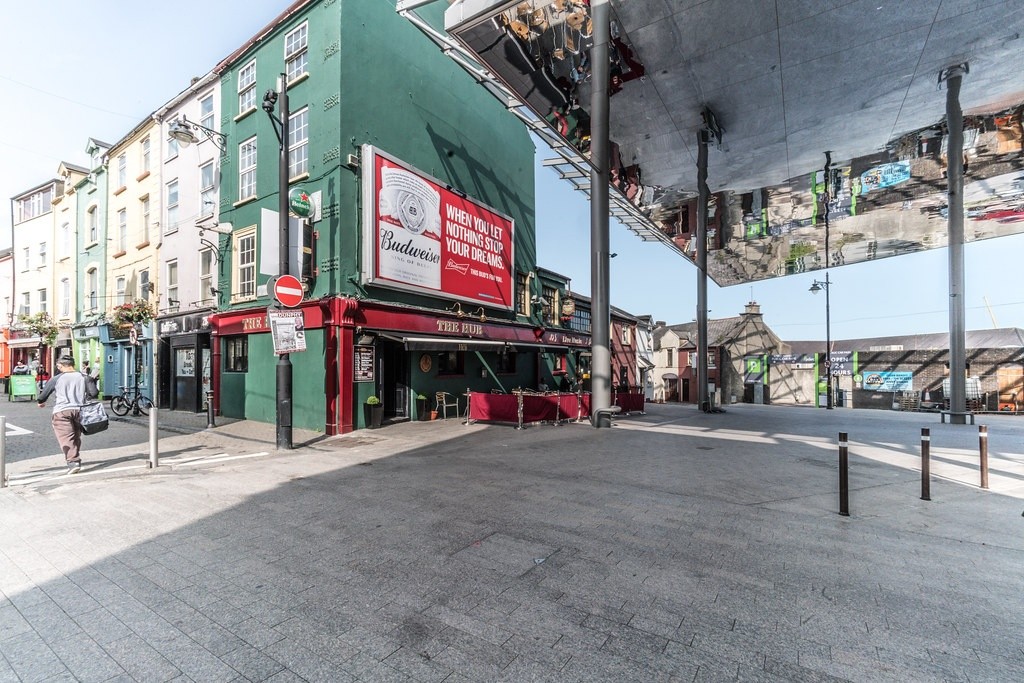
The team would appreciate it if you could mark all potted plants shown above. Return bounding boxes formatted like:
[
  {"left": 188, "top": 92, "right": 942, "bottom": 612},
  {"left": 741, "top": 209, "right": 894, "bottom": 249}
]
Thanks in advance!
[
  {"left": 364, "top": 395, "right": 383, "bottom": 430},
  {"left": 416, "top": 392, "right": 433, "bottom": 421}
]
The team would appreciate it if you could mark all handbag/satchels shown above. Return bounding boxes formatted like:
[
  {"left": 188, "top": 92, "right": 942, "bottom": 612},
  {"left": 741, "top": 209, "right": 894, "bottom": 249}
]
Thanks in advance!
[{"left": 78, "top": 396, "right": 108, "bottom": 435}]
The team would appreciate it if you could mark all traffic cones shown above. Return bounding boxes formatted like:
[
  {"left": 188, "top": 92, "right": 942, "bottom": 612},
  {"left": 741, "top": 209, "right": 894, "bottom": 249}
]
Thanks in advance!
[{"left": 656, "top": 390, "right": 666, "bottom": 404}]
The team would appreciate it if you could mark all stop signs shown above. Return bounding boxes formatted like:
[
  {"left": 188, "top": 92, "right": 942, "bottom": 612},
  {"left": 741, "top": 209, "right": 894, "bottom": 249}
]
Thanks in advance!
[{"left": 274, "top": 275, "right": 304, "bottom": 307}]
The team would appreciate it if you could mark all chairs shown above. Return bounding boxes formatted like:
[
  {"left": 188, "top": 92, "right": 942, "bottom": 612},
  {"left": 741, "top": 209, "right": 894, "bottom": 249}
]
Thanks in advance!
[
  {"left": 463, "top": 392, "right": 477, "bottom": 418},
  {"left": 435, "top": 392, "right": 459, "bottom": 419}
]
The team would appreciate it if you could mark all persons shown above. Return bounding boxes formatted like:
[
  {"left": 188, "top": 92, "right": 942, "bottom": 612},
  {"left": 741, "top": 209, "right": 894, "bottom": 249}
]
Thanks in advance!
[
  {"left": 12, "top": 355, "right": 50, "bottom": 399},
  {"left": 37, "top": 355, "right": 99, "bottom": 475}
]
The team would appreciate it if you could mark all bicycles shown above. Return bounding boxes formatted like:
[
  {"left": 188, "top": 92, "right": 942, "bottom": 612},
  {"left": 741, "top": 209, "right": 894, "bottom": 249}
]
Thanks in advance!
[{"left": 110, "top": 381, "right": 155, "bottom": 416}]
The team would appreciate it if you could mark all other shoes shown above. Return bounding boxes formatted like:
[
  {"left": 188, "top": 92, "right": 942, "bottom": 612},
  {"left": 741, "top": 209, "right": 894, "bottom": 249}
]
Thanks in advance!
[{"left": 67, "top": 461, "right": 80, "bottom": 474}]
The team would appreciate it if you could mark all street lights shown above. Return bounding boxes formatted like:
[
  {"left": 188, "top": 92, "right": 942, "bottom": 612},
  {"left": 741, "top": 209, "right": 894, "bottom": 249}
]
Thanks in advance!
[{"left": 808, "top": 271, "right": 834, "bottom": 409}]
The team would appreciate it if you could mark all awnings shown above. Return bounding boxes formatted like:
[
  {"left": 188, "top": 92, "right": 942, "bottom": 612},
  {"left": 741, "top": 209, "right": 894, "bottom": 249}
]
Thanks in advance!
[{"left": 365, "top": 328, "right": 569, "bottom": 354}]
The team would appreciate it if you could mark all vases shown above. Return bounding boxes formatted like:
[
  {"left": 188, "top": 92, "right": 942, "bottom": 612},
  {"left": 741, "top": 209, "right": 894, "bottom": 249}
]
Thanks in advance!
[
  {"left": 431, "top": 409, "right": 438, "bottom": 420},
  {"left": 125, "top": 316, "right": 143, "bottom": 322}
]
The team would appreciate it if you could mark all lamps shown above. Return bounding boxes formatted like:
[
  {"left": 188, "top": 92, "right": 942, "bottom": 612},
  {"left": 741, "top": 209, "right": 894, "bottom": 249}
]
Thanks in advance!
[
  {"left": 210, "top": 287, "right": 223, "bottom": 297},
  {"left": 468, "top": 307, "right": 487, "bottom": 322},
  {"left": 168, "top": 298, "right": 180, "bottom": 306},
  {"left": 168, "top": 114, "right": 228, "bottom": 156},
  {"left": 445, "top": 302, "right": 465, "bottom": 318}
]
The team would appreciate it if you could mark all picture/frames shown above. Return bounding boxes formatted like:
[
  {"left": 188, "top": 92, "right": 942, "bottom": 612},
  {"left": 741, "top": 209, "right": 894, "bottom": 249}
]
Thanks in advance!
[{"left": 419, "top": 353, "right": 433, "bottom": 373}]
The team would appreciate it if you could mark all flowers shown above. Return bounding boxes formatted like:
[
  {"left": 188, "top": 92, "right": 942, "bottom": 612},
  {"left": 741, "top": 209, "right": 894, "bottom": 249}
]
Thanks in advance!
[{"left": 113, "top": 296, "right": 157, "bottom": 338}]
